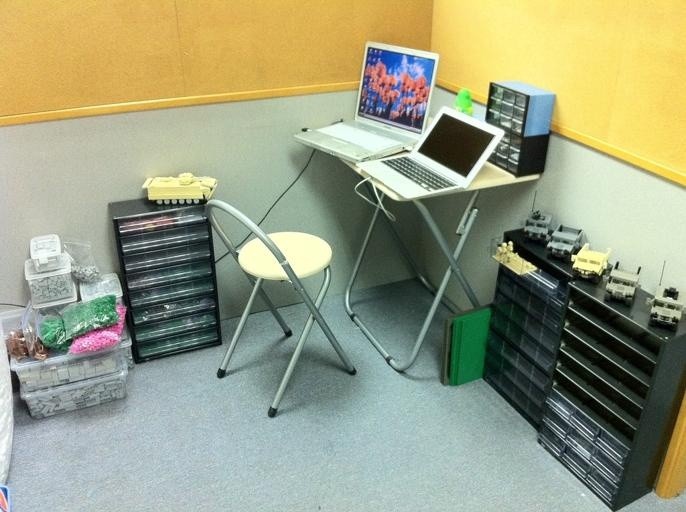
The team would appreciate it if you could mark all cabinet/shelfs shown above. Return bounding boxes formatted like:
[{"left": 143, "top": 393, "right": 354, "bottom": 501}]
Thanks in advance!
[{"left": 107, "top": 198, "right": 223, "bottom": 364}]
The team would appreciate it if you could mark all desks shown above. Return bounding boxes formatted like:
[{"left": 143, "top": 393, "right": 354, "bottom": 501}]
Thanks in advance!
[{"left": 339, "top": 115, "right": 540, "bottom": 371}]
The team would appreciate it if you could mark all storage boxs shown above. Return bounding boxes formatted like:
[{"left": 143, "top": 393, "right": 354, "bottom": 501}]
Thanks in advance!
[
  {"left": 19, "top": 357, "right": 127, "bottom": 420},
  {"left": 23, "top": 233, "right": 123, "bottom": 316},
  {"left": 11, "top": 333, "right": 121, "bottom": 393}
]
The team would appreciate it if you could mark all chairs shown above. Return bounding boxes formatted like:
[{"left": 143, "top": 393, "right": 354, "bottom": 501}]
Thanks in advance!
[{"left": 204, "top": 199, "right": 356, "bottom": 418}]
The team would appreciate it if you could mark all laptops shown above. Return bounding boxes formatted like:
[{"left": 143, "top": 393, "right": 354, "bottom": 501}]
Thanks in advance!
[
  {"left": 293, "top": 39, "right": 441, "bottom": 166},
  {"left": 355, "top": 105, "right": 506, "bottom": 203}
]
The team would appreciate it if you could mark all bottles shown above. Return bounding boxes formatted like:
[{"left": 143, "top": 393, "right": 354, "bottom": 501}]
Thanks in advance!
[{"left": 455, "top": 88, "right": 475, "bottom": 114}]
